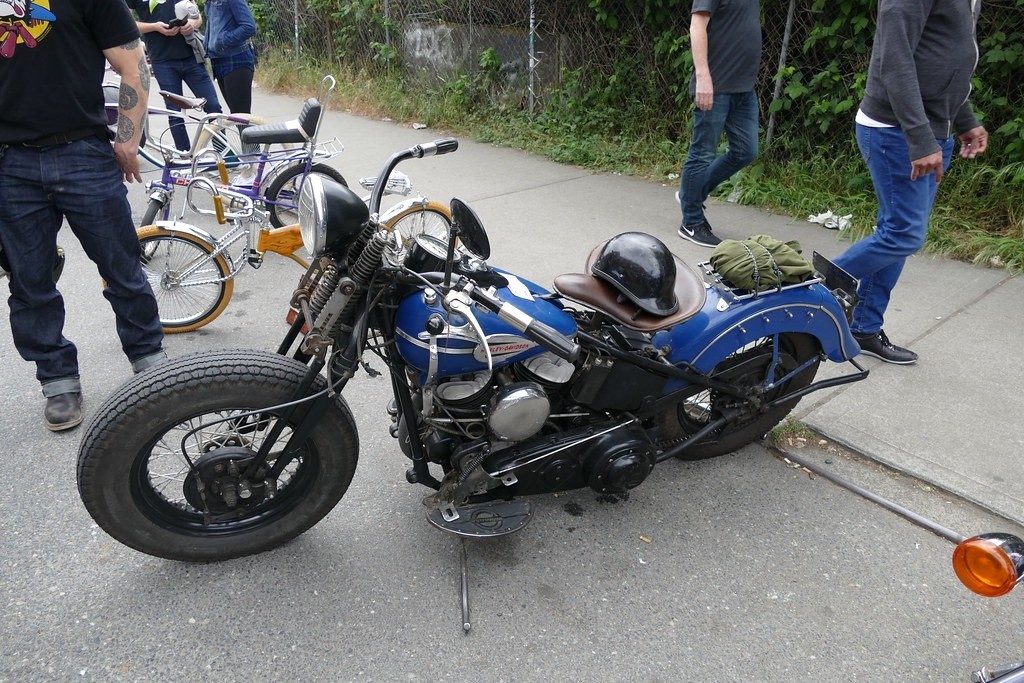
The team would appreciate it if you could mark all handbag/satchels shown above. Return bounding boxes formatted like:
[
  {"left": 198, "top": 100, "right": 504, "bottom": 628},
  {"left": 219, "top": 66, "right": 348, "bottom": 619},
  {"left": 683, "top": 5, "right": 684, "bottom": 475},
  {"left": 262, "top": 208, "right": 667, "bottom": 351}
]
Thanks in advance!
[{"left": 248, "top": 43, "right": 258, "bottom": 64}]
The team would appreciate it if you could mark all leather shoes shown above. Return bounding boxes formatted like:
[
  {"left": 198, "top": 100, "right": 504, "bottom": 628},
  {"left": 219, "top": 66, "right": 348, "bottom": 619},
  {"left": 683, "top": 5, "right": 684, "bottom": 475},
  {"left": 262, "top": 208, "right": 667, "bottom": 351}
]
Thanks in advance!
[
  {"left": 41, "top": 377, "right": 83, "bottom": 430},
  {"left": 132, "top": 349, "right": 168, "bottom": 375}
]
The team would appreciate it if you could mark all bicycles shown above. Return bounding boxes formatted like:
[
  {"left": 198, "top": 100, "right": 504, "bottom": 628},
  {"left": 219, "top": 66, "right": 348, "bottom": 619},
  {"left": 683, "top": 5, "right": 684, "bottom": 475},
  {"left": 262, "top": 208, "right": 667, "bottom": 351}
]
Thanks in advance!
[{"left": 99, "top": 64, "right": 462, "bottom": 333}]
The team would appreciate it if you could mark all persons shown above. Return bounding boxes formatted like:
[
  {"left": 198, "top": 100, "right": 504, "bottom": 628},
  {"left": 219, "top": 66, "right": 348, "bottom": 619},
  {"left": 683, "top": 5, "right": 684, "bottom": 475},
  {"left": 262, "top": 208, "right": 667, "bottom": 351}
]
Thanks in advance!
[
  {"left": 124, "top": 0, "right": 246, "bottom": 177},
  {"left": 203, "top": 0, "right": 257, "bottom": 187},
  {"left": 674, "top": 0, "right": 762, "bottom": 247},
  {"left": 0, "top": 0, "right": 170, "bottom": 432},
  {"left": 813, "top": 0, "right": 988, "bottom": 365}
]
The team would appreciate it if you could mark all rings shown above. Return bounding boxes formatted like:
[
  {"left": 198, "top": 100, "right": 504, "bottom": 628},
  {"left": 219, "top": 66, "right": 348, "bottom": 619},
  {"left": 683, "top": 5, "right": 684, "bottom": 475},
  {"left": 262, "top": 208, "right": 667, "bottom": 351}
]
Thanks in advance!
[{"left": 968, "top": 144, "right": 971, "bottom": 146}]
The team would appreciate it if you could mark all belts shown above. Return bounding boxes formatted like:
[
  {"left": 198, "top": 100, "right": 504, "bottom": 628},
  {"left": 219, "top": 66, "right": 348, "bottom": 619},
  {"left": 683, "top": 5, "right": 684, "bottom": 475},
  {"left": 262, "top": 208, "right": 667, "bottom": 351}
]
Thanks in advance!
[{"left": 23, "top": 127, "right": 95, "bottom": 147}]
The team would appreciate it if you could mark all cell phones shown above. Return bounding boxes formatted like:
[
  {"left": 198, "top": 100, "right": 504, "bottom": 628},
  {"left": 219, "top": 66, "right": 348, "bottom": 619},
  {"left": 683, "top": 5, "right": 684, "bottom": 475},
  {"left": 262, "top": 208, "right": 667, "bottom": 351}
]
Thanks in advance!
[{"left": 169, "top": 18, "right": 180, "bottom": 29}]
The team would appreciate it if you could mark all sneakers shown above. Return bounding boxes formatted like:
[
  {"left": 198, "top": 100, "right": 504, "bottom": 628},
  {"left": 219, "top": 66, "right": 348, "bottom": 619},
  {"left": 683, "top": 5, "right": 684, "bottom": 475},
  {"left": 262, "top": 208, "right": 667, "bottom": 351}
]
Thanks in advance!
[
  {"left": 678, "top": 219, "right": 721, "bottom": 248},
  {"left": 852, "top": 329, "right": 918, "bottom": 364},
  {"left": 232, "top": 173, "right": 256, "bottom": 185},
  {"left": 675, "top": 191, "right": 706, "bottom": 210}
]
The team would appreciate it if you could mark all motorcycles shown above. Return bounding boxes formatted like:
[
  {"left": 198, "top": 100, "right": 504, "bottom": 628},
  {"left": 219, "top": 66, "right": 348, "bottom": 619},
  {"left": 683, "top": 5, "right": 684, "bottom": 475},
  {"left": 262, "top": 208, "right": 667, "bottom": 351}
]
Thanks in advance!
[{"left": 76, "top": 137, "right": 870, "bottom": 634}]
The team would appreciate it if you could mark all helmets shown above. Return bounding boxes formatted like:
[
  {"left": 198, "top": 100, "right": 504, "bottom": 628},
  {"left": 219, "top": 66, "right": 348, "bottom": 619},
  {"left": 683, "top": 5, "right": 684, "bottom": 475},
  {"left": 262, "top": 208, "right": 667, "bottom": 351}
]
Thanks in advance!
[{"left": 591, "top": 232, "right": 680, "bottom": 316}]
